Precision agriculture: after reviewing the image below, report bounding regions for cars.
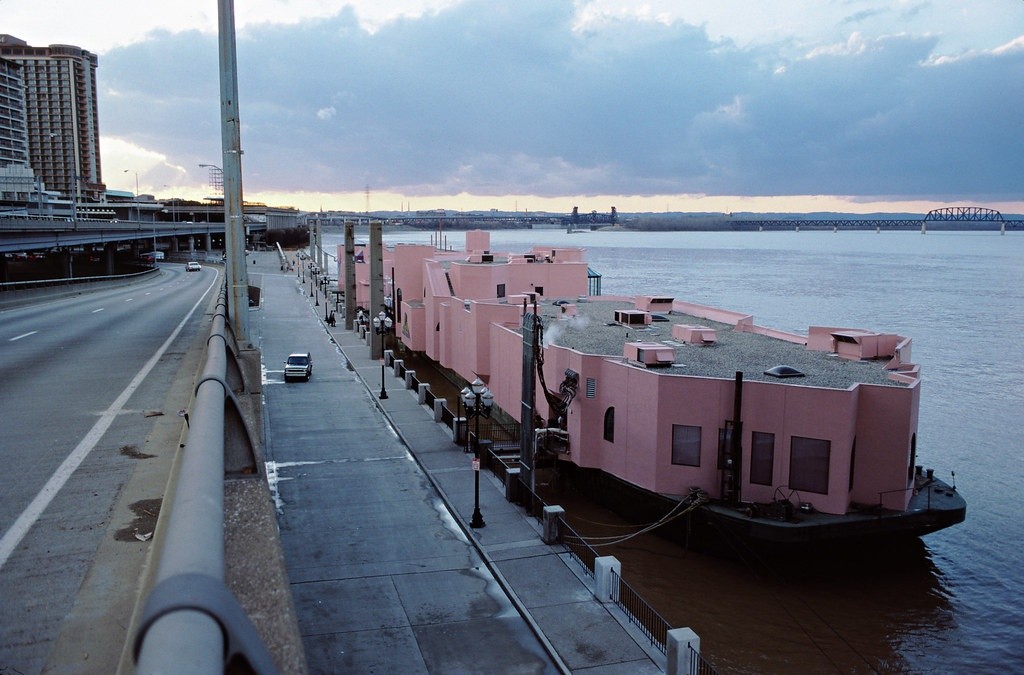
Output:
[184,262,202,272]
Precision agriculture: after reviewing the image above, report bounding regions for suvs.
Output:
[283,352,312,382]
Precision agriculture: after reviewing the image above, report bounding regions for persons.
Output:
[384,306,393,321]
[290,359,300,364]
[298,358,304,365]
[329,310,336,327]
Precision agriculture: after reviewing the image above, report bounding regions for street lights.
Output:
[308,262,315,297]
[373,311,393,399]
[164,184,175,223]
[124,169,142,220]
[50,133,77,222]
[319,274,331,321]
[299,254,307,283]
[152,209,168,264]
[295,251,302,277]
[460,375,495,529]
[312,267,321,306]
